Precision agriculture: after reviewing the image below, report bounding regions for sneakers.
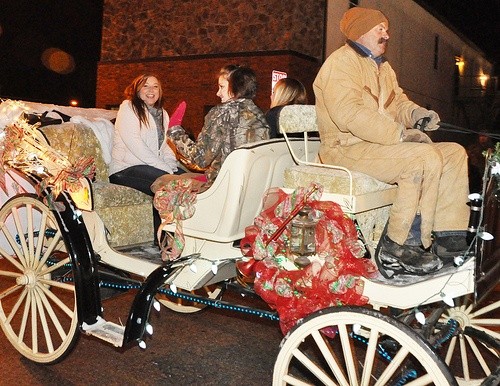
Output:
[379,249,437,274]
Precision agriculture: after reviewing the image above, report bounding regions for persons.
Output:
[108,71,188,250]
[263,78,317,138]
[312,7,474,273]
[152,64,270,193]
[466,129,494,192]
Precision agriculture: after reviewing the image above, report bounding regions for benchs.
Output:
[162,137,321,242]
[279,105,399,214]
[39,123,155,248]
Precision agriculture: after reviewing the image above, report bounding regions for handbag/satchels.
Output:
[155,179,197,220]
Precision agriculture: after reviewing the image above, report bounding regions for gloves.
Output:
[168,101,187,128]
[402,129,432,144]
[412,107,440,131]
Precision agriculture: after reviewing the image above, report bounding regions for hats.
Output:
[340,8,390,43]
